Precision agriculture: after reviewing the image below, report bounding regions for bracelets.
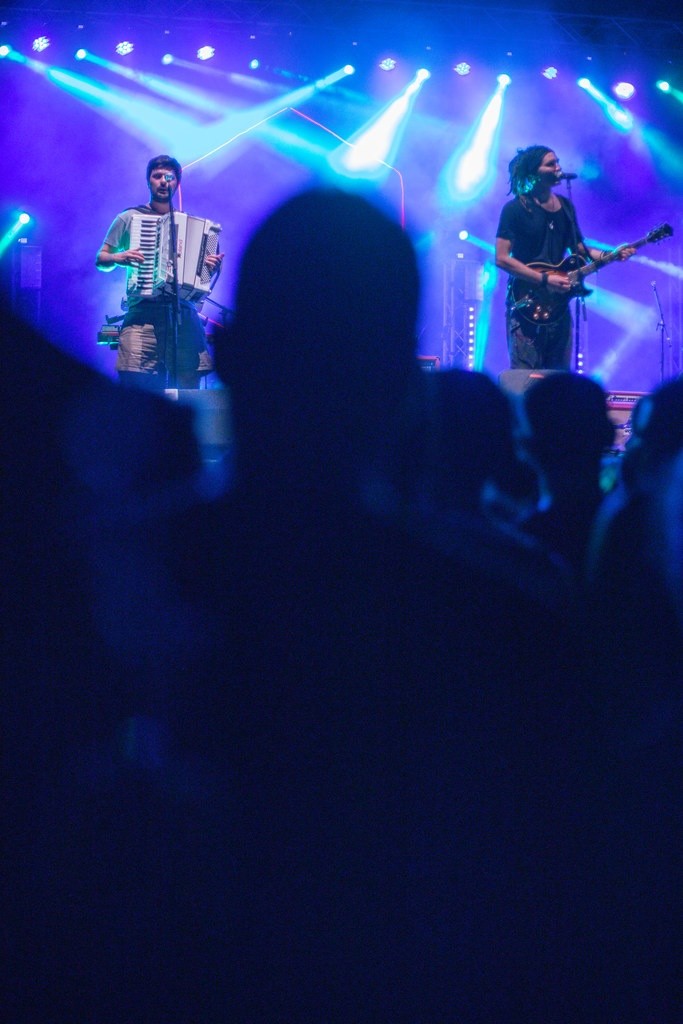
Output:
[542,274,549,284]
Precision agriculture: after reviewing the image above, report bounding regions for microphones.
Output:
[557,172,577,179]
[164,172,174,181]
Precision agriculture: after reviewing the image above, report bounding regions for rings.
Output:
[559,284,562,288]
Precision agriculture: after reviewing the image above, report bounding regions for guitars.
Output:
[511,220,676,325]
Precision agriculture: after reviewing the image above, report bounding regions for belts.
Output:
[141,296,195,310]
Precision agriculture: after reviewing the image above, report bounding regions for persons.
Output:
[95,154,226,399]
[494,144,638,371]
[0,190,683,1021]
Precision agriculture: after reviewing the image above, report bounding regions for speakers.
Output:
[164,388,233,460]
[605,390,650,452]
[497,369,561,397]
